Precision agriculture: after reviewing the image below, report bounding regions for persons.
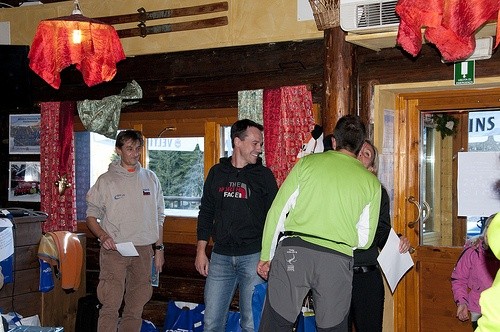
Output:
[85,130,166,332]
[256,115,500,332]
[193,118,278,332]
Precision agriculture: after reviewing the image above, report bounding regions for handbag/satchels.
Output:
[140,319,158,332]
[296,296,317,332]
[164,300,205,332]
[225,311,242,332]
[252,281,268,332]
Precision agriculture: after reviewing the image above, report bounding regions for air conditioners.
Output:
[340,0,400,35]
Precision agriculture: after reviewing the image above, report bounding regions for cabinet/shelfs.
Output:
[0,215,86,332]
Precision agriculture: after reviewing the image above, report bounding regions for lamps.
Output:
[27,0,126,90]
[395,0,500,62]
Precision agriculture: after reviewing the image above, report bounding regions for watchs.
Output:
[155,244,164,251]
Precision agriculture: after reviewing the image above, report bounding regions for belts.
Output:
[354,266,377,274]
